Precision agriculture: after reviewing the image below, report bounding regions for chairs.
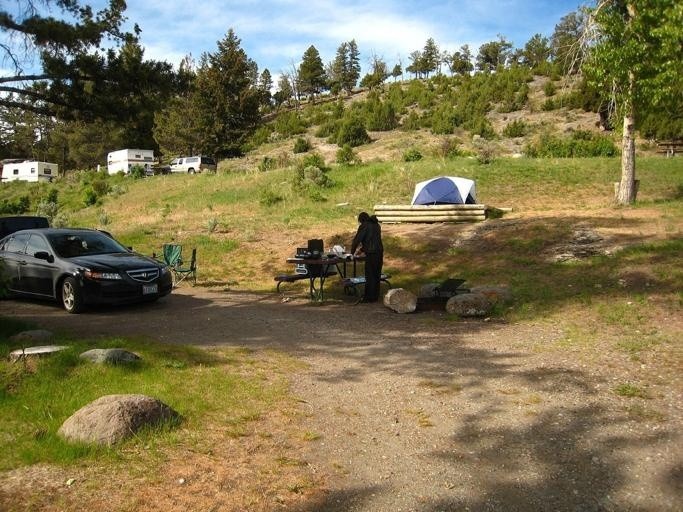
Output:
[173,248,197,289]
[151,243,182,286]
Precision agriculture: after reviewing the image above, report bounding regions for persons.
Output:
[349,211,384,303]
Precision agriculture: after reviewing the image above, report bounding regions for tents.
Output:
[410,175,477,204]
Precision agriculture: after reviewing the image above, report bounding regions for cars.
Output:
[170,156,217,174]
[0,227,171,314]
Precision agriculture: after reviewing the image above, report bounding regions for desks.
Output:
[287,256,366,303]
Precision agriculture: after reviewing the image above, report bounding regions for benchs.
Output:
[337,274,392,299]
[275,272,337,293]
